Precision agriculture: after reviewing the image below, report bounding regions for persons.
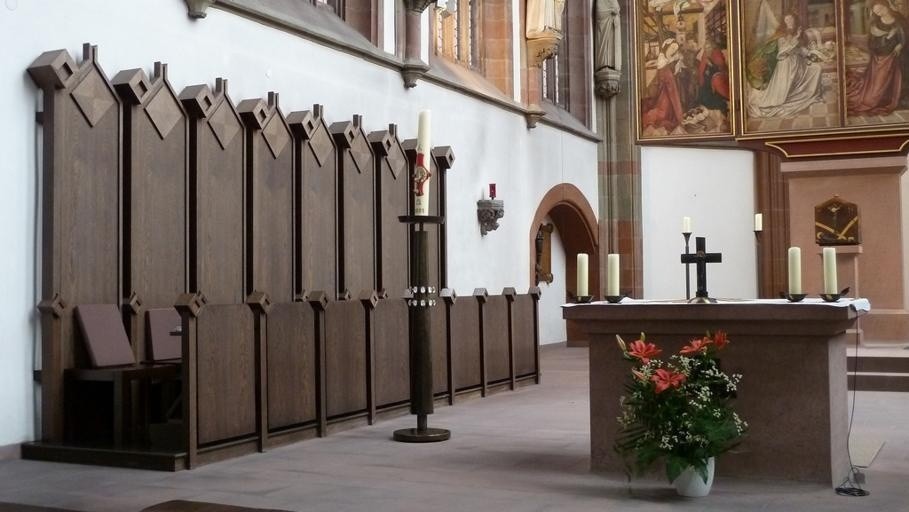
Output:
[637,28,730,130]
[846,0,908,117]
[746,9,825,122]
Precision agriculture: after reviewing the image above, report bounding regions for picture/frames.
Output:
[728,0,844,140]
[835,0,909,134]
[624,1,739,146]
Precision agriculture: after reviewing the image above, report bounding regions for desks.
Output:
[556,292,873,488]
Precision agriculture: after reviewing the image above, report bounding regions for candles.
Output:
[414,105,431,218]
[787,246,804,295]
[823,246,839,295]
[683,215,691,234]
[576,251,590,297]
[606,252,620,296]
[489,183,497,197]
[755,213,762,233]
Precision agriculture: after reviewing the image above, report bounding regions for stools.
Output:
[64,363,175,456]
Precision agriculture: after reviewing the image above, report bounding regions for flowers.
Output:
[594,317,760,485]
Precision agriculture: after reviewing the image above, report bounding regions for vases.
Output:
[667,452,716,498]
